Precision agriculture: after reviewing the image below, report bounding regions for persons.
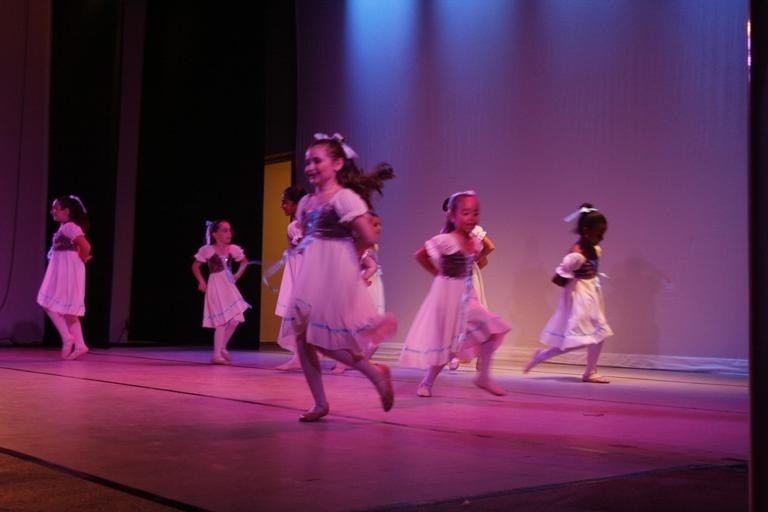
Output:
[523,203,614,384]
[36,195,92,360]
[191,219,252,363]
[274,133,396,421]
[397,191,510,395]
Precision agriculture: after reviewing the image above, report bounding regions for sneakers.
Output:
[221,348,231,361]
[583,372,610,383]
[373,365,392,412]
[68,345,87,360]
[416,382,431,397]
[472,378,506,396]
[298,405,329,421]
[211,357,225,363]
[61,337,74,360]
[448,362,457,369]
[275,345,377,374]
[522,351,540,372]
